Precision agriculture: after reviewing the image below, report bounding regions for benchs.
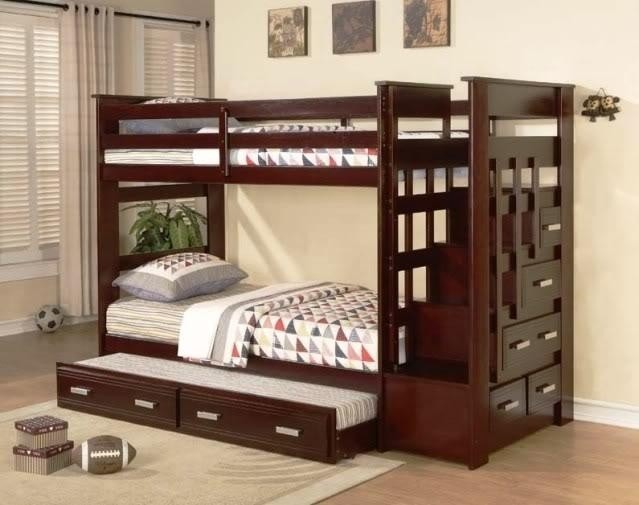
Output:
[55,347,379,465]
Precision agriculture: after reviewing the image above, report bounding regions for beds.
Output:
[89,90,498,471]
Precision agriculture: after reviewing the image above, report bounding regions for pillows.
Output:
[110,249,250,303]
[116,95,241,134]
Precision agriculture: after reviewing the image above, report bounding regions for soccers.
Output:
[34,304,65,334]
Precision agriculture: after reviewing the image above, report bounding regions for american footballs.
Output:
[69,435,137,475]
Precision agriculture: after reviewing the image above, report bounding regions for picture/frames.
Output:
[266,6,309,58]
[331,0,378,55]
[402,0,452,48]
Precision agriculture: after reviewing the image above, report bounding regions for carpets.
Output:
[1,396,407,505]
